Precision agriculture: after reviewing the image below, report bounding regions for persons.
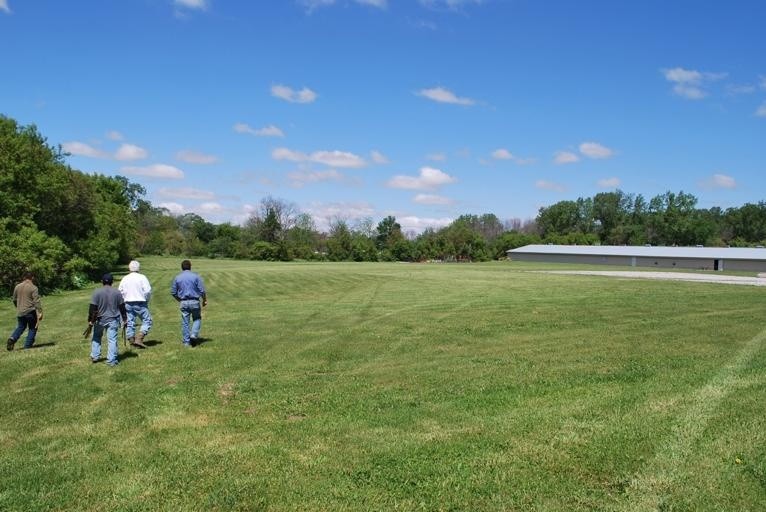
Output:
[118,260,152,348]
[6,272,43,352]
[171,259,207,346]
[88,272,128,366]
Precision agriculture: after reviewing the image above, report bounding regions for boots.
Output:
[128,332,148,349]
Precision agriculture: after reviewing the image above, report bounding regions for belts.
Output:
[180,298,199,301]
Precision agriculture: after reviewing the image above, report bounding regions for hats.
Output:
[102,273,113,282]
[129,260,140,272]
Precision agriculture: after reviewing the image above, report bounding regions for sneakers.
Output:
[7,337,14,351]
